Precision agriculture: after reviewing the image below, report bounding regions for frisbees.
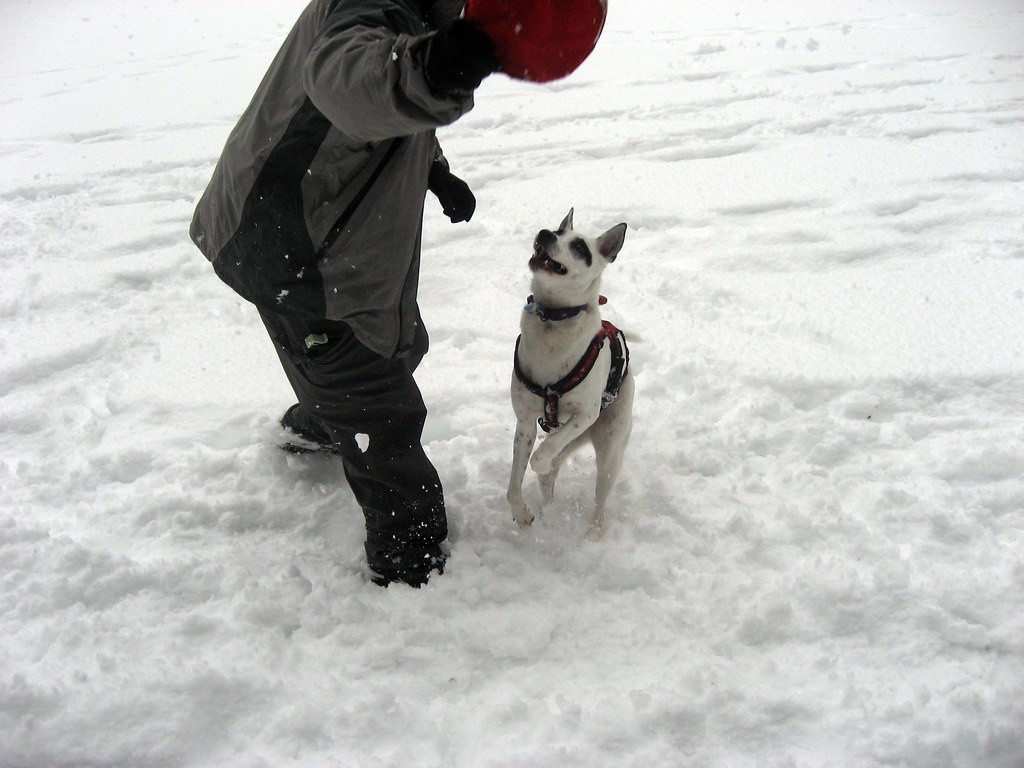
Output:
[466,0,608,84]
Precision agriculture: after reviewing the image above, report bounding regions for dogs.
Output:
[505,205,647,545]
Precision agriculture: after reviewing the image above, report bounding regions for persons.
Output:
[187,0,504,588]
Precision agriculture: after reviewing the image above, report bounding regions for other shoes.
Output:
[276,402,340,455]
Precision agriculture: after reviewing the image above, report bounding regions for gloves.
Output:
[437,173,476,223]
[422,18,504,90]
